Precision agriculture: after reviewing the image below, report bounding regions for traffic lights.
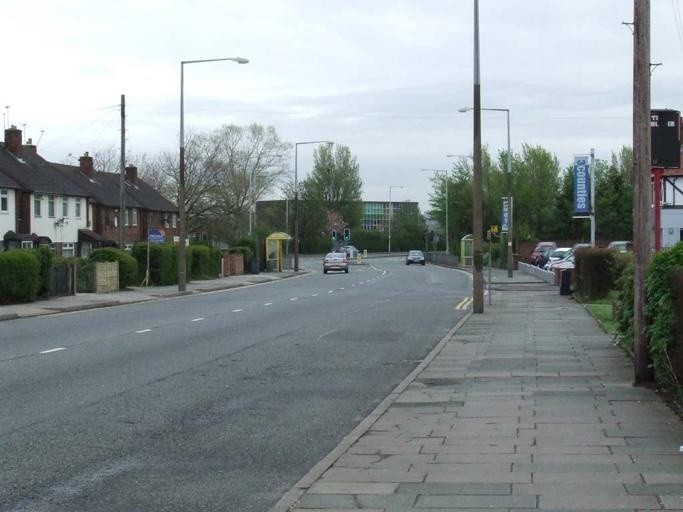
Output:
[344,228,351,240]
[332,230,337,240]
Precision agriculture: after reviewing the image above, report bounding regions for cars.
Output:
[405,250,425,265]
[323,252,348,274]
[530,240,632,272]
[341,246,359,256]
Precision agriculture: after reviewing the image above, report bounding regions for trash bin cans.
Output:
[251,258,260,274]
[560,269,571,295]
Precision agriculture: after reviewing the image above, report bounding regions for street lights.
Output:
[178,56,249,292]
[388,185,404,253]
[457,107,514,278]
[294,140,335,272]
[446,154,473,159]
[249,154,283,235]
[421,168,449,255]
